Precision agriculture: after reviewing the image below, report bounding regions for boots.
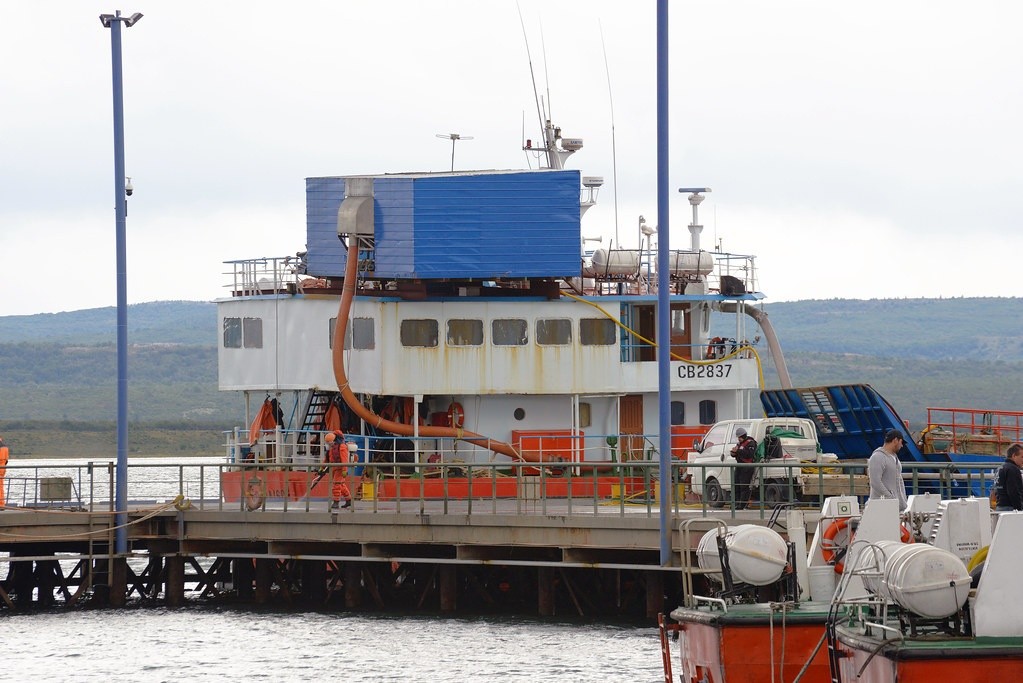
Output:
[341,499,351,508]
[331,501,339,508]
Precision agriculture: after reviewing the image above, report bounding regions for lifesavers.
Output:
[447,402,465,429]
[706,336,722,361]
[823,519,863,574]
[243,476,265,510]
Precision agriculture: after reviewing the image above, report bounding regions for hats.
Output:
[885,429,908,446]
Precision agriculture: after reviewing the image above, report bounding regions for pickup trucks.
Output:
[684,415,868,503]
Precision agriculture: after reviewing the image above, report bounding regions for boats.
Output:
[214,4,1021,506]
[655,490,939,683]
[824,490,1022,683]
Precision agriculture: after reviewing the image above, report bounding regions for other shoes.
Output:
[731,504,748,510]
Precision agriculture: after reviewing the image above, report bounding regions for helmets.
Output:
[325,433,336,442]
[736,428,746,437]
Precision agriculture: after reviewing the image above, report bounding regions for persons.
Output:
[313,432,352,509]
[0,439,9,508]
[868,430,908,511]
[728,428,758,509]
[994,443,1023,512]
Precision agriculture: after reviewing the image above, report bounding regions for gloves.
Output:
[342,471,347,478]
[318,467,323,474]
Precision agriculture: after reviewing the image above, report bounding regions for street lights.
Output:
[101,9,145,605]
